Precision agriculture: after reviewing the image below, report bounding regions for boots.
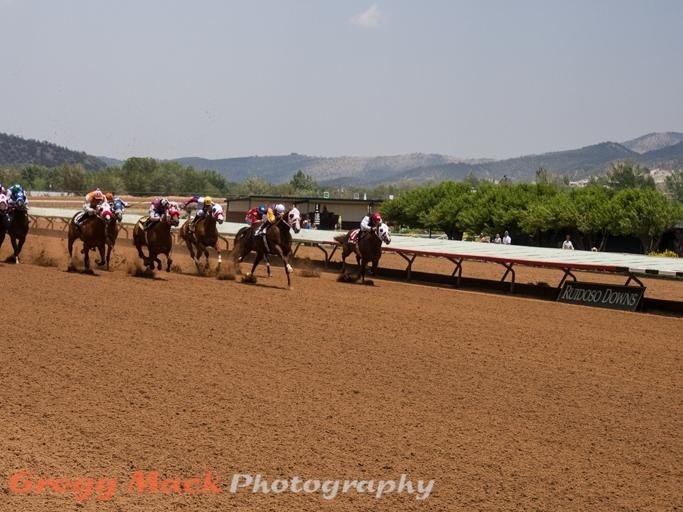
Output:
[76,212,89,227]
[258,223,266,235]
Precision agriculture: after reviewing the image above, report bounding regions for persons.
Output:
[0,182,30,222]
[302,218,317,231]
[74,190,130,233]
[288,204,300,222]
[476,230,511,263]
[560,234,576,250]
[333,222,343,230]
[347,211,382,243]
[148,194,287,236]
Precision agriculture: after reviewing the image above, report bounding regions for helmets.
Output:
[258,204,266,215]
[106,192,113,201]
[276,204,285,214]
[204,196,212,206]
[162,199,169,208]
[14,184,21,192]
[370,213,381,225]
[94,190,103,200]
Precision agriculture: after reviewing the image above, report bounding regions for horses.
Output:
[233,204,302,291]
[65,202,113,273]
[179,203,227,277]
[77,198,129,270]
[0,185,29,265]
[132,200,181,273]
[333,220,391,286]
